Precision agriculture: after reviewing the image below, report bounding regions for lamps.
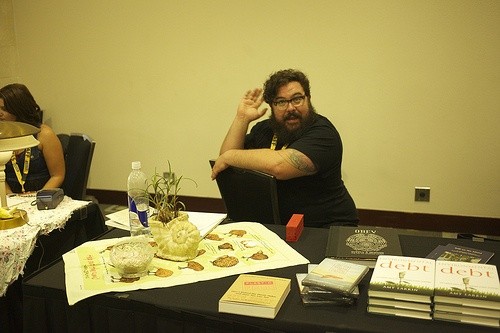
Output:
[0,121,40,230]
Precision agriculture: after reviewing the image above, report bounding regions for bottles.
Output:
[127,162,150,237]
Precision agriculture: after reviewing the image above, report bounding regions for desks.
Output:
[0,195,500,333]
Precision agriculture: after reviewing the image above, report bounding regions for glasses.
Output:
[271,93,306,106]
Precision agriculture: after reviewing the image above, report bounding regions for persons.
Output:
[0,83,66,197]
[211,69,359,229]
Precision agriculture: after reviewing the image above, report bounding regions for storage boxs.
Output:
[286,214,304,242]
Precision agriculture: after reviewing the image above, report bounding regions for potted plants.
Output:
[126,159,200,262]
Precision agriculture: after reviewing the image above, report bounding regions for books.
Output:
[366,242,500,329]
[325,225,403,268]
[296,257,369,305]
[105,204,158,232]
[219,273,291,320]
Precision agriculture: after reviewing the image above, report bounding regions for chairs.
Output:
[57,132,96,201]
[208,160,279,225]
